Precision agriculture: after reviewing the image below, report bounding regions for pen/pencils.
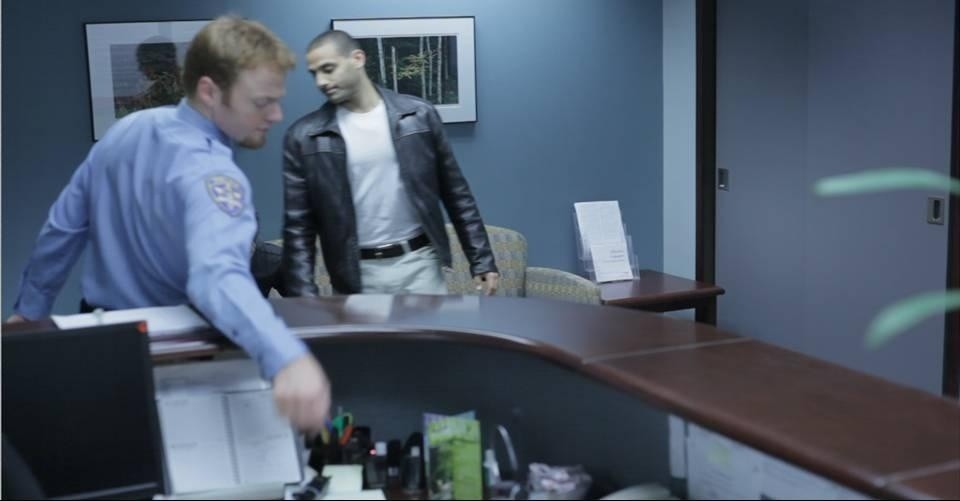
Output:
[93,307,105,326]
[320,406,353,446]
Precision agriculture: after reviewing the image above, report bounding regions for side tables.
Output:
[593,268,726,329]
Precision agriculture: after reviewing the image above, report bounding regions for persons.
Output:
[129,33,184,110]
[280,31,500,300]
[7,11,334,438]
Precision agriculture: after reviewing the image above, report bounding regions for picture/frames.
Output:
[84,18,219,145]
[330,15,478,125]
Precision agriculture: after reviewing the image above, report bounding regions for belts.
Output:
[359,233,430,261]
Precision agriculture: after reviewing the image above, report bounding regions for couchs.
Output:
[264,220,603,312]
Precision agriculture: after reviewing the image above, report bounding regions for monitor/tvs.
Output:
[2,319,174,501]
